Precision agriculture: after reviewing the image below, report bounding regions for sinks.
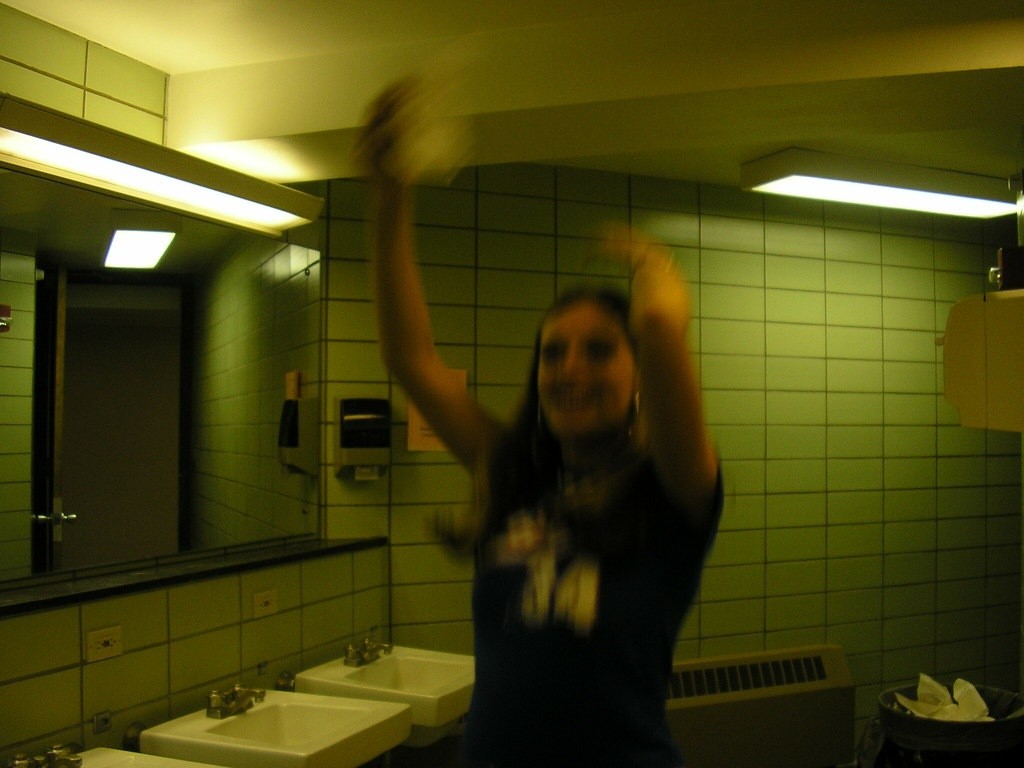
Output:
[137,686,414,767]
[73,745,224,767]
[290,640,480,751]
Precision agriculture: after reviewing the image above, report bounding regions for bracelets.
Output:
[630,246,663,273]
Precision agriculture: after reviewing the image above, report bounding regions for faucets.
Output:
[205,683,267,721]
[343,635,392,668]
[8,740,84,768]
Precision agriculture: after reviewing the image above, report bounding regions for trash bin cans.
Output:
[855,680,1024,768]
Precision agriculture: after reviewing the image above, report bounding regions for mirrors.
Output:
[0,168,320,583]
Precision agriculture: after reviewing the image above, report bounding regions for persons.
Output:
[355,72,724,768]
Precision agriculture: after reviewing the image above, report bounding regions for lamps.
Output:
[741,147,1017,220]
[0,91,326,239]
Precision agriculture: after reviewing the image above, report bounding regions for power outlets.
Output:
[253,589,278,621]
[85,625,122,663]
